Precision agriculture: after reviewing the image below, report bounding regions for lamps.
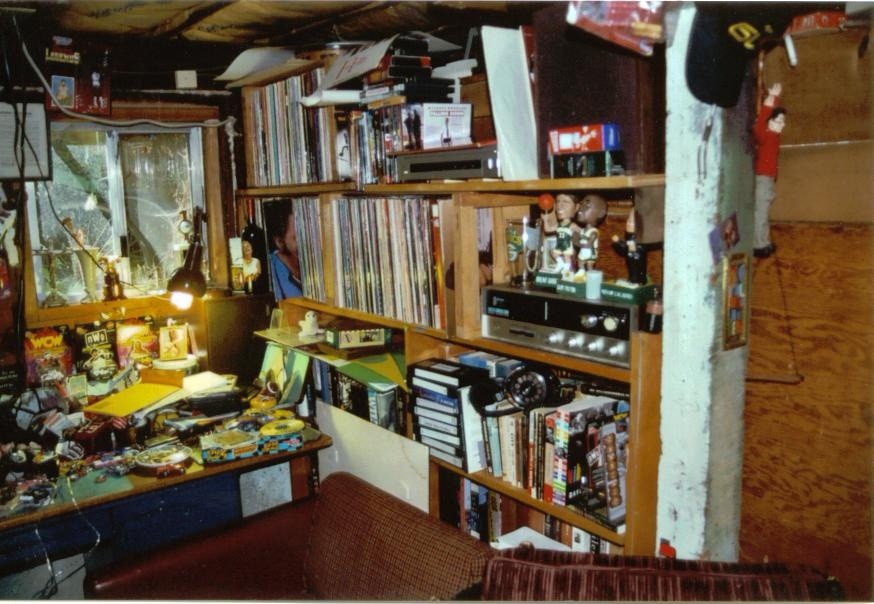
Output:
[167,205,233,311]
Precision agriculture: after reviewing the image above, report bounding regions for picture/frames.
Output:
[720,252,749,350]
[159,324,187,361]
[0,97,54,183]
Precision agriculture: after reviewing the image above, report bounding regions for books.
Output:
[307,358,404,435]
[247,40,472,188]
[248,197,493,331]
[406,357,630,558]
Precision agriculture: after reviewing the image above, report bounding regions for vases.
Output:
[71,247,103,304]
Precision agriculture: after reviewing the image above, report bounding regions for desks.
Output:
[0,382,336,579]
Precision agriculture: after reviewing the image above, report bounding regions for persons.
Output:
[541,192,608,284]
[264,200,303,300]
[752,82,787,259]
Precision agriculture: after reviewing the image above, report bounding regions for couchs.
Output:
[484,552,847,602]
[85,471,499,600]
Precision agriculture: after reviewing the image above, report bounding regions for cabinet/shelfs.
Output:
[239,54,665,560]
